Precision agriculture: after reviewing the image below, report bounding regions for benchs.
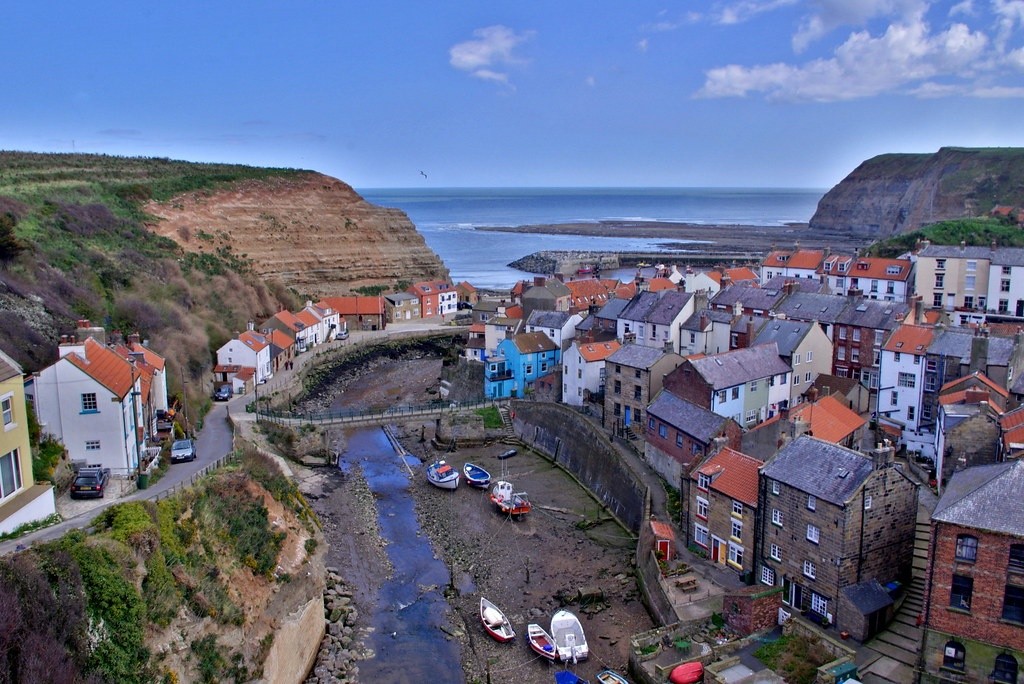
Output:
[682,585,699,593]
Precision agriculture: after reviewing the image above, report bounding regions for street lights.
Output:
[182,373,188,439]
[131,392,141,473]
[201,366,204,392]
[255,384,262,423]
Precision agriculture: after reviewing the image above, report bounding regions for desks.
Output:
[677,575,696,587]
[677,641,691,655]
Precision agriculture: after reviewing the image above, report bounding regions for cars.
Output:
[70,467,109,500]
[170,439,196,464]
[457,302,474,309]
[215,385,232,401]
[336,332,349,340]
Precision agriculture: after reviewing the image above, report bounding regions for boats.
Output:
[596,671,628,684]
[554,670,590,684]
[528,624,556,660]
[490,458,531,514]
[480,596,516,643]
[578,261,593,275]
[636,263,651,268]
[427,460,459,488]
[550,609,588,664]
[670,661,704,684]
[463,463,491,488]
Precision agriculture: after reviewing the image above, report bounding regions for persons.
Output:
[974,321,989,338]
[284,361,289,370]
[289,360,294,370]
[938,320,947,327]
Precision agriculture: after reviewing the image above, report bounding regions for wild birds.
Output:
[420,171,427,179]
